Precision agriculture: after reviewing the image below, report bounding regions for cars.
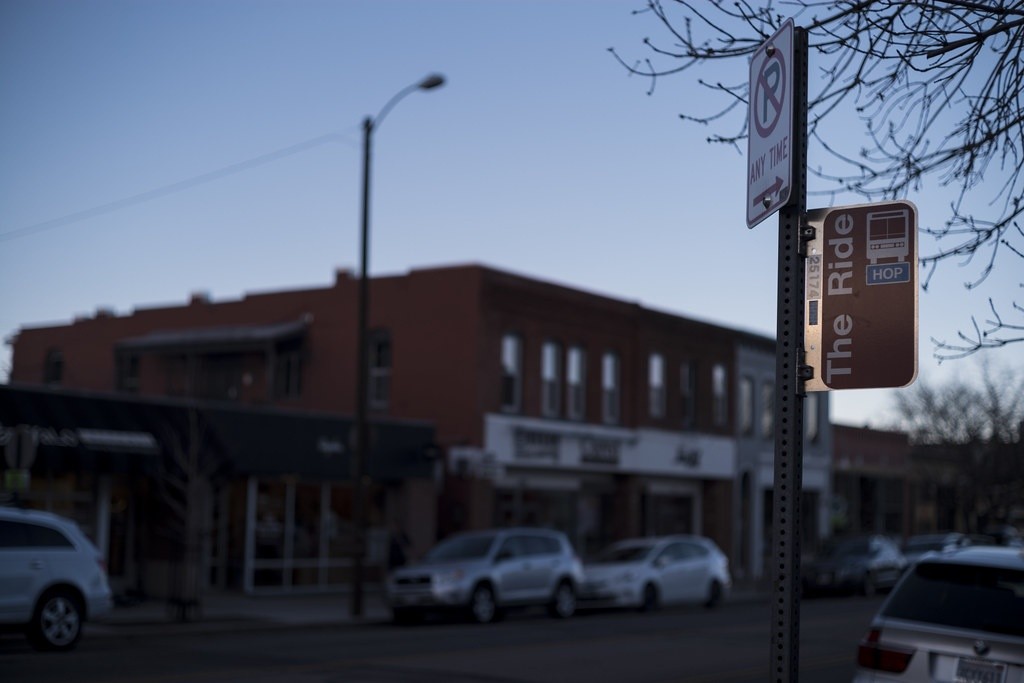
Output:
[584,535,731,618]
[798,526,1024,594]
[388,525,591,624]
[0,506,115,653]
[851,546,1024,683]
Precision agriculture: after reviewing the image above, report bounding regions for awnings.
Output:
[0,385,436,483]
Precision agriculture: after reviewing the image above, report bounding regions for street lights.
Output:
[349,72,446,616]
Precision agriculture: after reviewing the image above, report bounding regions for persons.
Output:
[381,519,411,586]
